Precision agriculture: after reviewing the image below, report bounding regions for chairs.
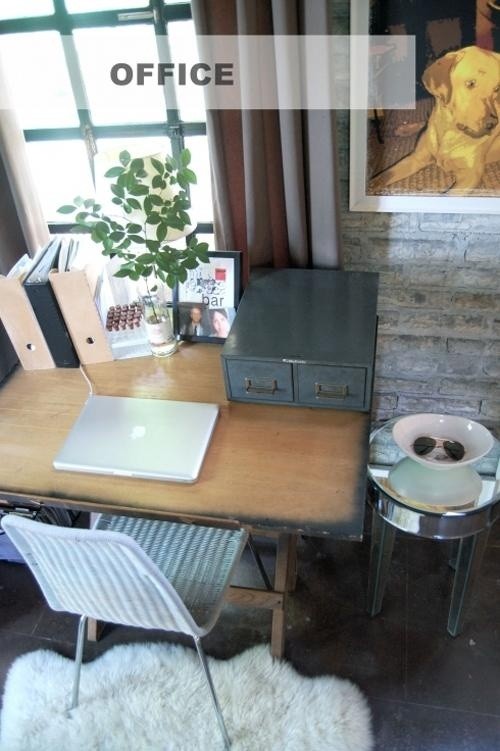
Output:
[0,513,273,751]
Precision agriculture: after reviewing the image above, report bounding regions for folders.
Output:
[0,237,114,371]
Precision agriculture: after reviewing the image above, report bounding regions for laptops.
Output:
[53,395,219,485]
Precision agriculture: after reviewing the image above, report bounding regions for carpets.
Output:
[0,644,377,751]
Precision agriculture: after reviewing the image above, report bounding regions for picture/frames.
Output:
[172,250,241,344]
[350,0,500,215]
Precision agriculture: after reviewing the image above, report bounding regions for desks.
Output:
[0,315,377,663]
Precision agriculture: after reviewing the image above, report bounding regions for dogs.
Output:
[368,44,499,197]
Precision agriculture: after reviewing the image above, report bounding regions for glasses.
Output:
[414,438,464,460]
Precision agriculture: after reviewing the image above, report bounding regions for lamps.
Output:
[93,146,197,307]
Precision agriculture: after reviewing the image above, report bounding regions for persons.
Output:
[208,309,231,338]
[183,307,206,336]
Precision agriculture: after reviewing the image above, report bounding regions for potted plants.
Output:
[56,148,211,358]
